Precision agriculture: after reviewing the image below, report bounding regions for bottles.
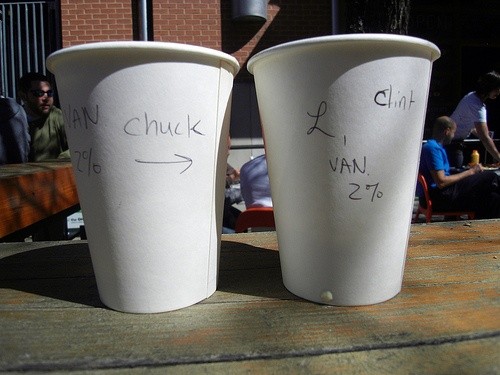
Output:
[471,150,480,165]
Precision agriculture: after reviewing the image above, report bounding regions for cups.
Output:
[246,34,441,306]
[45,41,240,313]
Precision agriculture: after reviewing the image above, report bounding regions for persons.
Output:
[444,73,500,171]
[17,71,74,162]
[221,136,245,232]
[415,117,500,220]
[239,155,277,231]
[0,96,31,165]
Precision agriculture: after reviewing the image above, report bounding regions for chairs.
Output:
[234,207,275,234]
[414,175,476,224]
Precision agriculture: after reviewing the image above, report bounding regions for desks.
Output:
[1,159,79,242]
[0,219,499,375]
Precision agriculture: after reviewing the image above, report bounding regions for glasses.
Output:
[30,88,53,97]
